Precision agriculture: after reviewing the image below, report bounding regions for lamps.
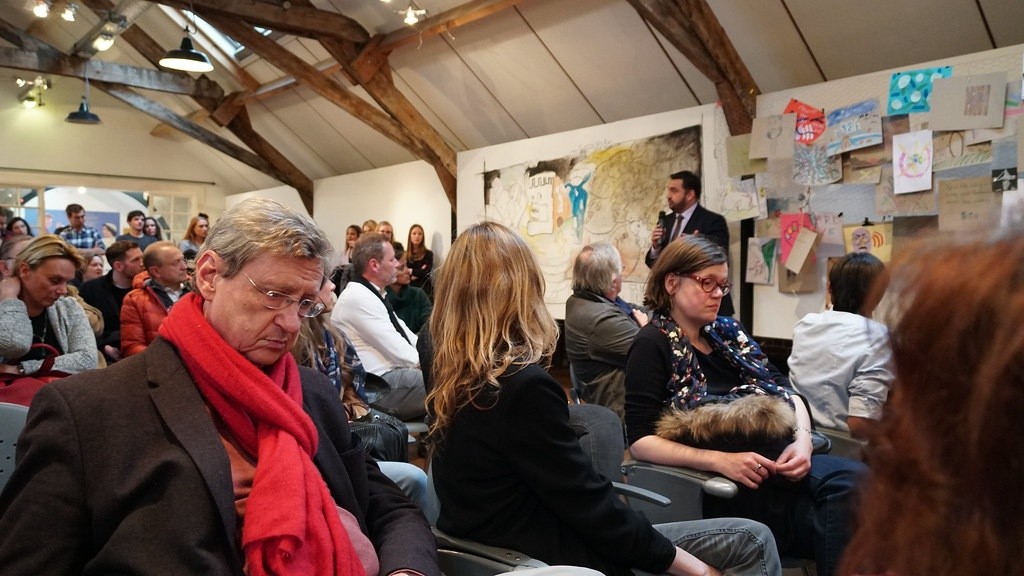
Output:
[33,0,55,17]
[91,33,114,51]
[16,76,52,107]
[392,1,426,25]
[60,4,80,22]
[64,76,102,124]
[158,24,214,73]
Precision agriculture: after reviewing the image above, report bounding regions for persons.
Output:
[644,170,735,318]
[0,196,440,576]
[300,273,439,526]
[625,234,868,576]
[425,221,782,576]
[838,223,1024,576]
[330,234,430,421]
[0,203,211,377]
[564,242,654,448]
[787,252,897,438]
[337,219,433,333]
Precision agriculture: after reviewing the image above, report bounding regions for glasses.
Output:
[165,258,188,266]
[240,270,327,317]
[686,275,732,296]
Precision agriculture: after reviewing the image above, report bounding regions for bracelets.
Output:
[792,427,813,442]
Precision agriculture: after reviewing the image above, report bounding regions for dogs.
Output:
[654,393,796,450]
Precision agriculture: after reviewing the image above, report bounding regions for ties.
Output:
[672,215,684,241]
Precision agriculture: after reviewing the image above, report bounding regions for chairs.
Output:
[0,402,31,493]
[432,360,873,576]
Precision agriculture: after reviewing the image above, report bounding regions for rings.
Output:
[755,464,761,473]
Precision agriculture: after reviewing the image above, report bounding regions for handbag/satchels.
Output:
[0,344,68,407]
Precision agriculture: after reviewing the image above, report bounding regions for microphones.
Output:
[657,211,665,244]
